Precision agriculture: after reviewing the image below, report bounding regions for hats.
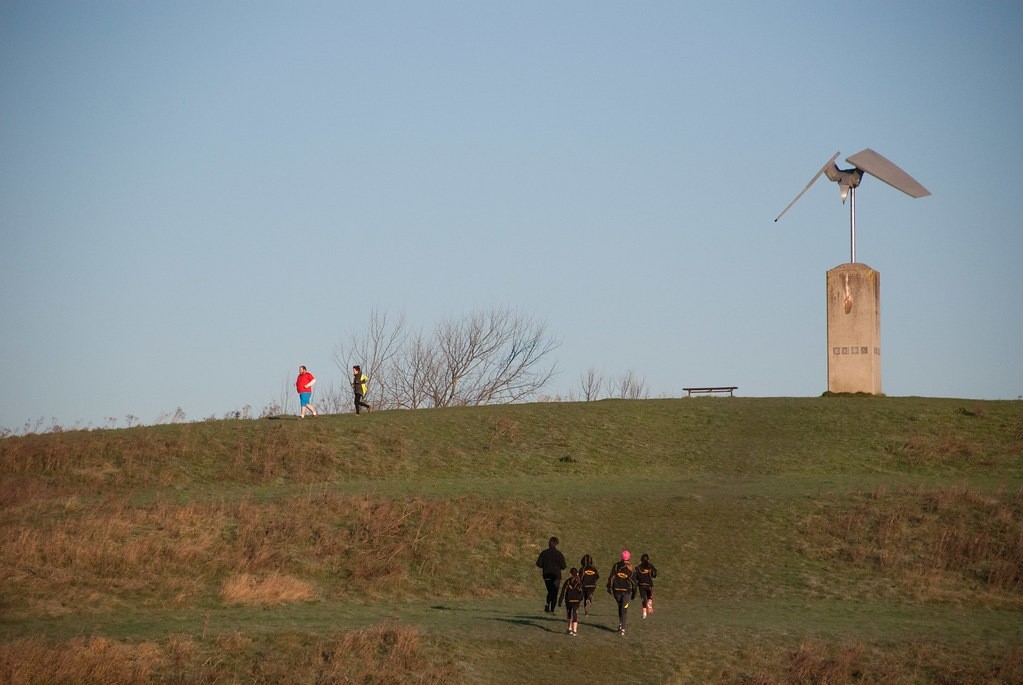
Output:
[621,551,631,561]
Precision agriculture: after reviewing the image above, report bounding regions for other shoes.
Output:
[618,624,626,635]
[550,611,557,616]
[585,613,589,618]
[566,629,573,635]
[367,405,372,413]
[312,411,318,417]
[586,599,591,608]
[296,416,304,420]
[352,414,360,416]
[573,632,578,636]
[545,604,547,613]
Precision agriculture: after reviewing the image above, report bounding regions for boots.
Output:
[647,599,654,615]
[642,607,647,620]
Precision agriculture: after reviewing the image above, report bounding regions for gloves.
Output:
[631,590,637,600]
[606,587,611,593]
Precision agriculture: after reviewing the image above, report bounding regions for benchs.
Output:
[682,387,738,397]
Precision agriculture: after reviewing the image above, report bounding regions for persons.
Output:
[579,554,599,618]
[536,536,567,616]
[294,366,319,419]
[558,567,582,637]
[352,365,373,416]
[634,554,657,619]
[607,550,637,636]
